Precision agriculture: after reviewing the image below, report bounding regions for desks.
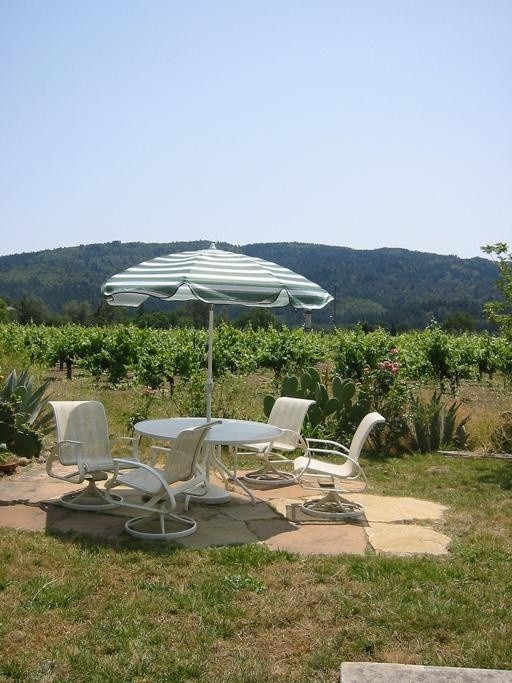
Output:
[134,418,283,511]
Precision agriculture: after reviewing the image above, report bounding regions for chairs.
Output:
[229,397,316,484]
[104,420,222,540]
[293,412,386,518]
[46,401,139,510]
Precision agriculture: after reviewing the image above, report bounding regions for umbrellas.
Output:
[100,241,334,485]
